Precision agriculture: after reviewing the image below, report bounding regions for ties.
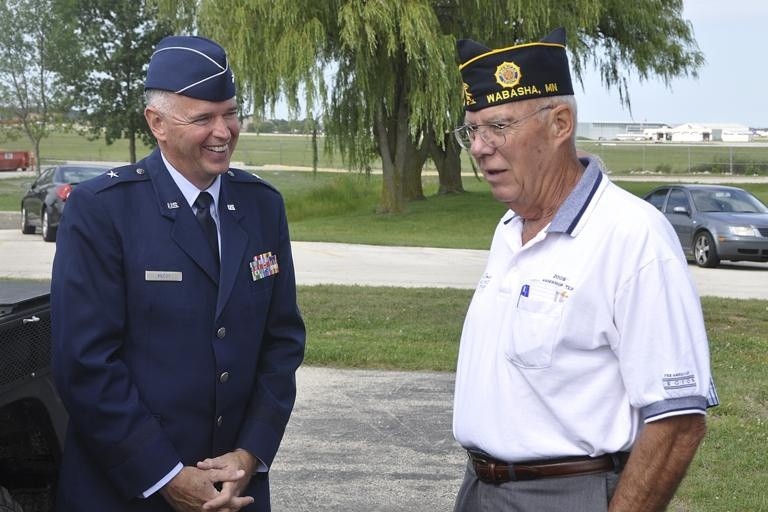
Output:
[191,191,224,274]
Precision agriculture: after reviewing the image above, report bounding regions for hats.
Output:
[452,24,574,112]
[143,33,235,102]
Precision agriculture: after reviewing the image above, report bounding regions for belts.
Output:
[466,444,626,485]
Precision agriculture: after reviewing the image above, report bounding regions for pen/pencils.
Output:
[515,284,531,307]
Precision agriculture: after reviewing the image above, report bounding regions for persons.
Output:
[453,27,719,512]
[50,37,305,512]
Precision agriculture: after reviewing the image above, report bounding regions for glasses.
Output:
[451,106,558,150]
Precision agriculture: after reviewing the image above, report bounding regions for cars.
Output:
[644,184,768,268]
[17,164,111,243]
